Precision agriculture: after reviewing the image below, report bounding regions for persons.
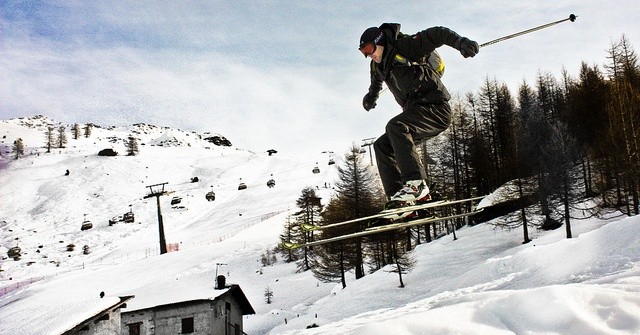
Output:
[359,22,480,225]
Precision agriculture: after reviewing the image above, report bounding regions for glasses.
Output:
[358,41,377,58]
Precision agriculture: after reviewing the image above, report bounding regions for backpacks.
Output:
[372,50,445,80]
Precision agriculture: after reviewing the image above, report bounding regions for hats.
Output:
[360,27,387,46]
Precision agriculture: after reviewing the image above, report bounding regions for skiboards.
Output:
[282,194,486,250]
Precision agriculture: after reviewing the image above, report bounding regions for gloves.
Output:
[363,88,379,112]
[456,37,479,58]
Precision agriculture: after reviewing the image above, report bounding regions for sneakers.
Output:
[385,178,432,209]
[378,209,419,223]
